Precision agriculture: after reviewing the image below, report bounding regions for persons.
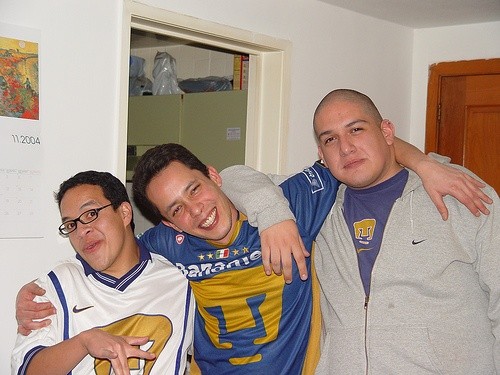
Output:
[215,89,499,374]
[15,136,493,374]
[10,170,198,375]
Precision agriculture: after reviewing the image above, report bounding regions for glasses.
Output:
[58,203,120,235]
